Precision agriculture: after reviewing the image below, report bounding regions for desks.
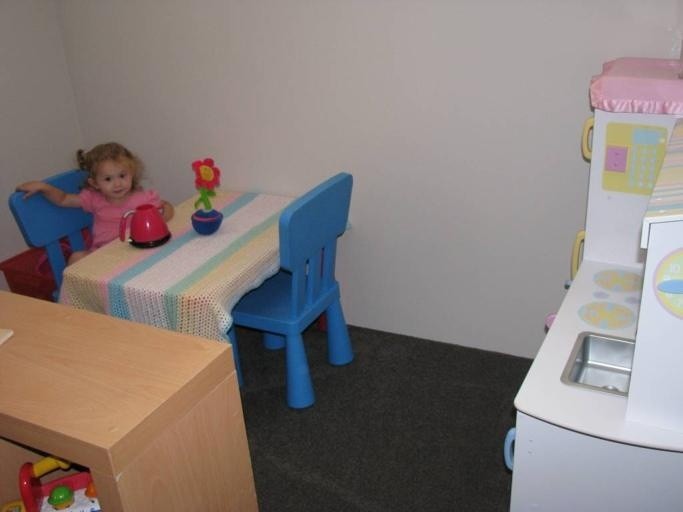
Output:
[62,191,297,342]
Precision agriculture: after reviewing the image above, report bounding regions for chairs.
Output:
[225,170,353,408]
[8,170,95,302]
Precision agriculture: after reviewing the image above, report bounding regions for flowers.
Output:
[190,158,223,212]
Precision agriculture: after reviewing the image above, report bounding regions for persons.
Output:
[14,142,175,265]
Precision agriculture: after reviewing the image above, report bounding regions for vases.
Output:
[191,208,224,236]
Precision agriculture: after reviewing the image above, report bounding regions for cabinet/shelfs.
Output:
[0,289,262,512]
